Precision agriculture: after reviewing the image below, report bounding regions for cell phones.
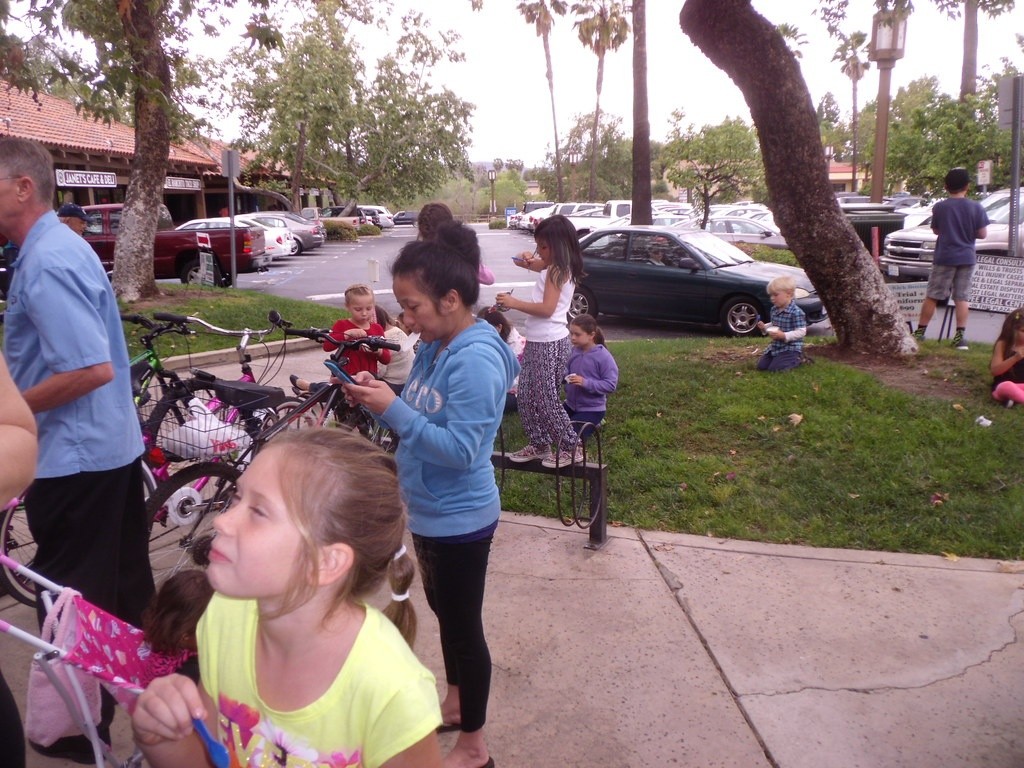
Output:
[324,360,357,385]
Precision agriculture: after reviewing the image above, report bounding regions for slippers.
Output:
[434,713,466,733]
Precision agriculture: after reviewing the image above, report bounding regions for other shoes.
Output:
[1003,398,1016,408]
[290,374,304,389]
[28,728,113,762]
[910,330,926,343]
[950,339,968,351]
[292,387,310,399]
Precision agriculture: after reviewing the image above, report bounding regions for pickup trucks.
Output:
[81,202,272,288]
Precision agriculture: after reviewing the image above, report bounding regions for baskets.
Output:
[128,378,270,461]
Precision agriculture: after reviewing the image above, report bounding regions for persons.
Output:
[323,284,391,435]
[290,305,415,398]
[0,137,156,765]
[142,569,214,684]
[132,430,444,768]
[562,314,618,438]
[341,222,522,768]
[57,202,95,237]
[477,307,525,415]
[394,311,422,354]
[494,215,588,468]
[758,276,814,372]
[912,169,990,350]
[647,243,664,265]
[0,355,38,768]
[988,307,1024,408]
[418,203,494,285]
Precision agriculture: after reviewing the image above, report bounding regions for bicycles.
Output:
[0,310,402,609]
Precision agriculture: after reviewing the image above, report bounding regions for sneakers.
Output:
[542,451,585,467]
[511,445,553,461]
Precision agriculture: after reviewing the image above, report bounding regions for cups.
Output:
[564,373,577,385]
[496,291,512,313]
[767,327,780,336]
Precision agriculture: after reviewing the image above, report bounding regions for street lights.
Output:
[487,170,496,217]
[867,9,908,203]
[823,142,833,178]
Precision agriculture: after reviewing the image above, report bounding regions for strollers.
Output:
[0,497,200,768]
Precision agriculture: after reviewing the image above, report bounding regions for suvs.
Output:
[878,186,1024,281]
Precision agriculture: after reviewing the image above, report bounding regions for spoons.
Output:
[193,717,229,768]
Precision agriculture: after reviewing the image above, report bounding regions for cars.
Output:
[566,224,829,338]
[508,191,993,250]
[174,205,421,260]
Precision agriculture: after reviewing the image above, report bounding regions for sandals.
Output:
[797,352,815,365]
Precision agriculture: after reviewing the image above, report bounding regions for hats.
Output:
[57,202,96,223]
[945,167,973,187]
